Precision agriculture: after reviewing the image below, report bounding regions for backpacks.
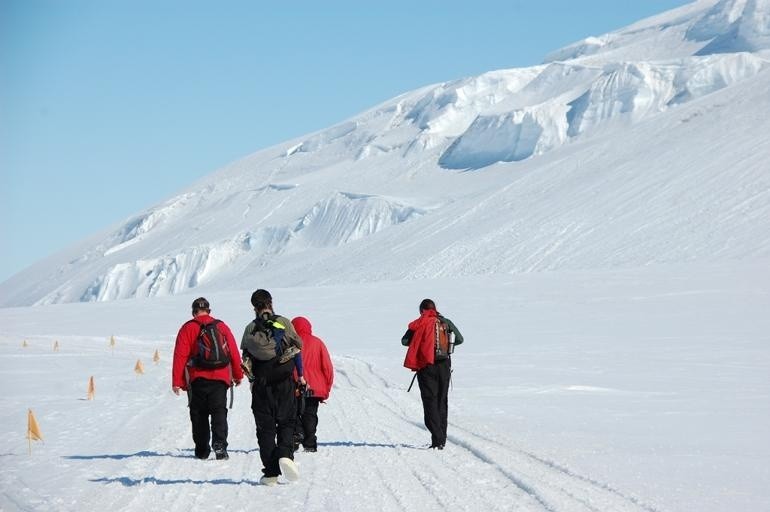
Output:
[192,319,230,370]
[433,316,455,360]
[241,315,302,383]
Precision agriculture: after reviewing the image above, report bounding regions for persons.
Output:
[401,299,463,449]
[290,316,333,452]
[240,289,310,486]
[172,297,243,460]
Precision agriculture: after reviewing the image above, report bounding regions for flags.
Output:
[153,350,160,364]
[134,359,144,375]
[27,412,43,440]
[86,375,95,398]
[110,336,116,347]
[54,340,61,351]
[22,341,27,348]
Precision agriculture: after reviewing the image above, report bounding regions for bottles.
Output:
[448,331,457,354]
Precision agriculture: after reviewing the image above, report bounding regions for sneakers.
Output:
[430,440,446,449]
[212,441,228,459]
[261,458,298,485]
[294,433,317,453]
